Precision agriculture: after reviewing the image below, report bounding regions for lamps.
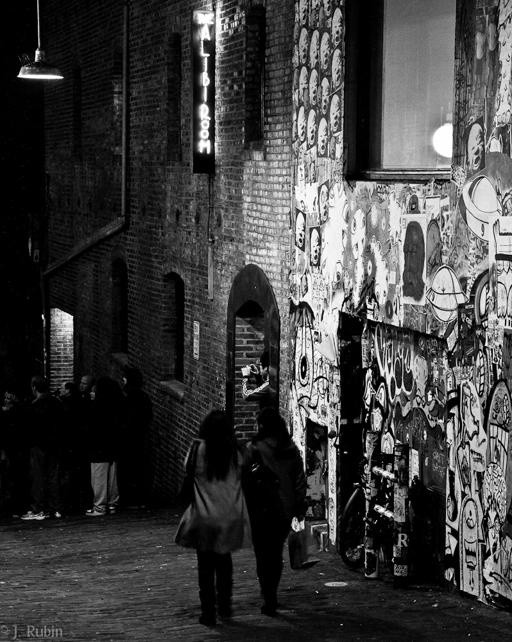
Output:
[17,1,65,82]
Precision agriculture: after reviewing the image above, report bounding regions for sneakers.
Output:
[109,505,116,513]
[260,603,278,610]
[85,509,107,517]
[44,511,62,519]
[21,511,45,521]
[199,615,216,625]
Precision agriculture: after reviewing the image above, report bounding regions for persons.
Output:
[241,412,308,616]
[0,370,152,522]
[174,411,252,629]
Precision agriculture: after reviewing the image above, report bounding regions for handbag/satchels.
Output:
[173,506,234,552]
[288,530,320,570]
